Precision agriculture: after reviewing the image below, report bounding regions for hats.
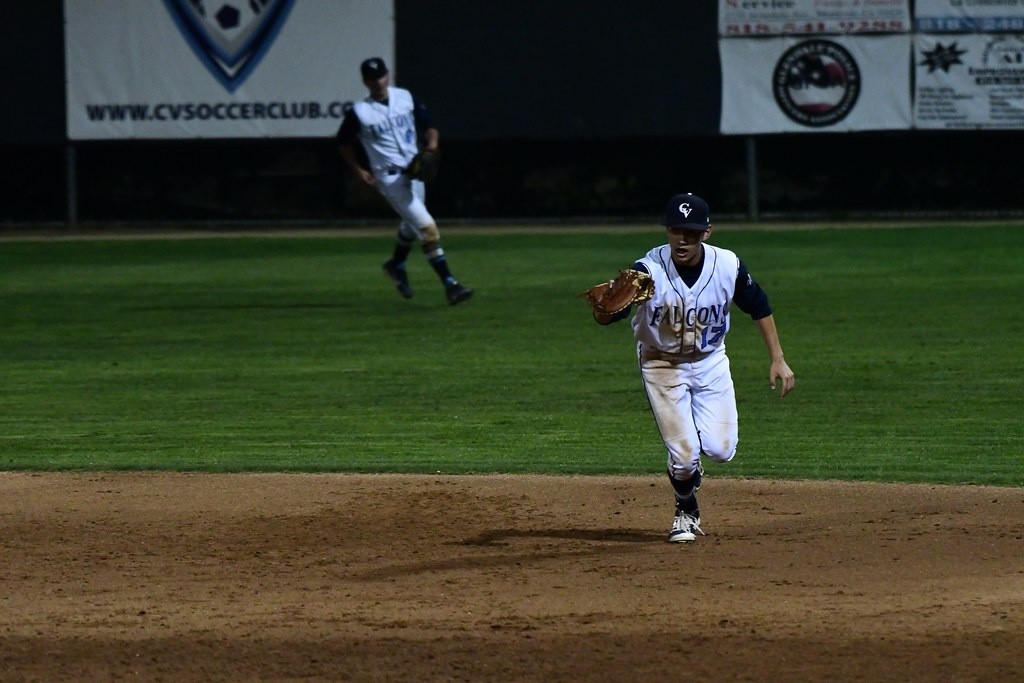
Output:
[660,194,709,230]
[361,57,389,77]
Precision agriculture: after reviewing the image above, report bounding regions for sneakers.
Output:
[668,508,705,542]
[693,460,704,490]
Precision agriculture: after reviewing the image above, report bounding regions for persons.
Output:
[593,194,795,544]
[336,58,474,306]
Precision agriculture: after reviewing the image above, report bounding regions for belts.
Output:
[645,347,714,364]
[388,169,406,176]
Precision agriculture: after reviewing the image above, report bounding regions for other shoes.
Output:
[384,261,412,299]
[446,280,472,305]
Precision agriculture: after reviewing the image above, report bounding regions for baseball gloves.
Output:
[403,149,442,182]
[582,268,656,316]
